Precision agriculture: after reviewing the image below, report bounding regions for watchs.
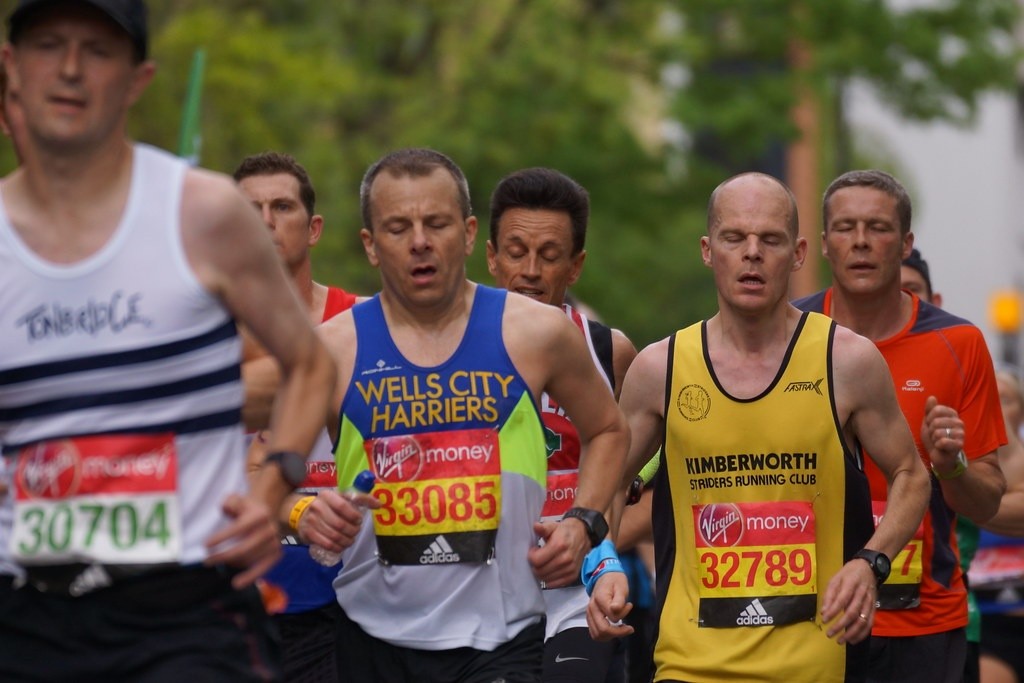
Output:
[563,508,609,548]
[847,549,891,586]
[266,451,306,489]
[626,476,644,505]
[930,452,967,481]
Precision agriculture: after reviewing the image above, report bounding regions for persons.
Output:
[0,0,340,683]
[227,153,1024,683]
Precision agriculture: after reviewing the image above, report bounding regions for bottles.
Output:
[309,470,375,567]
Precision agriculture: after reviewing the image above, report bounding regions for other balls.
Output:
[988,291,1023,334]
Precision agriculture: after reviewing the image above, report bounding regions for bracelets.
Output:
[288,496,316,532]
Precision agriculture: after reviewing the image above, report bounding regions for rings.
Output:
[541,581,546,590]
[946,429,952,438]
[860,614,869,622]
